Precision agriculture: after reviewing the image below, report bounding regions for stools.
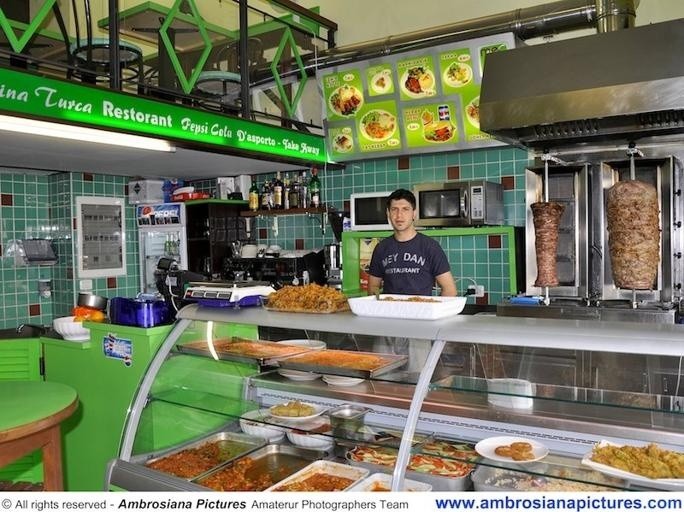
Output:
[70,38,146,99]
[196,69,244,112]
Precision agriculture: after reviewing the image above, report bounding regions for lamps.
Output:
[0,114,175,153]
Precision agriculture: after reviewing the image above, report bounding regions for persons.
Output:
[368,189,457,372]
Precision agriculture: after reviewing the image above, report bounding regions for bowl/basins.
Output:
[239,408,285,441]
[286,418,337,451]
[51,315,90,341]
[77,292,108,310]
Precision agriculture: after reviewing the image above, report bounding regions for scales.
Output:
[183,282,275,308]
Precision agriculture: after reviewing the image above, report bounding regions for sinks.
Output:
[0,326,42,339]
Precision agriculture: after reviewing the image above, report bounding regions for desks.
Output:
[0,383,78,490]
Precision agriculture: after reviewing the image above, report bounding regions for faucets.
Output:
[17,323,47,336]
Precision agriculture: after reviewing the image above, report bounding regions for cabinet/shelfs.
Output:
[40,323,258,491]
[342,226,523,298]
[241,205,338,222]
[108,305,684,493]
[185,200,249,276]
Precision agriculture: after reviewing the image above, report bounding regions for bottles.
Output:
[343,217,349,232]
[177,231,180,255]
[439,105,450,122]
[311,167,321,206]
[262,179,274,209]
[249,181,259,211]
[285,171,311,209]
[274,170,283,209]
[339,264,343,280]
[164,234,170,253]
[170,233,176,255]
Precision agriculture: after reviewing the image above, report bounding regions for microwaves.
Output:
[349,190,428,230]
[412,179,505,230]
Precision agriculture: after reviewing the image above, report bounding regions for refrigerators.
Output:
[135,202,188,300]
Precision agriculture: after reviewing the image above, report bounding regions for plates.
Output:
[262,306,350,316]
[331,133,353,155]
[261,459,369,491]
[345,428,484,490]
[358,109,397,143]
[421,120,457,144]
[328,84,363,118]
[347,294,467,320]
[370,73,392,94]
[470,453,630,491]
[474,436,549,465]
[175,336,316,365]
[240,243,281,258]
[189,443,329,490]
[321,374,364,387]
[464,96,480,128]
[274,339,326,381]
[143,431,269,483]
[344,471,433,490]
[267,401,327,420]
[399,65,435,100]
[581,438,684,491]
[443,62,472,88]
[274,348,408,379]
